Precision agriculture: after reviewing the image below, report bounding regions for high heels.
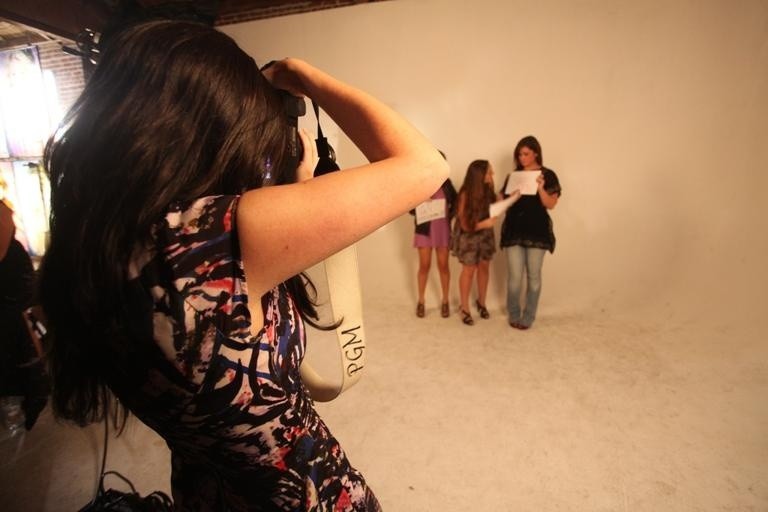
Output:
[475,299,489,319]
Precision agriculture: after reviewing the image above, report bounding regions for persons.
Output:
[449,160,504,325]
[410,151,457,317]
[500,136,561,330]
[0,201,49,431]
[36,17,451,512]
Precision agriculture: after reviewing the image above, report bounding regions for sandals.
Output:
[459,305,474,325]
[416,301,425,318]
[441,301,449,317]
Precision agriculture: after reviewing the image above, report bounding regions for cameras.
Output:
[259,86,306,190]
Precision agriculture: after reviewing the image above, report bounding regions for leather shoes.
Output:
[509,322,528,330]
